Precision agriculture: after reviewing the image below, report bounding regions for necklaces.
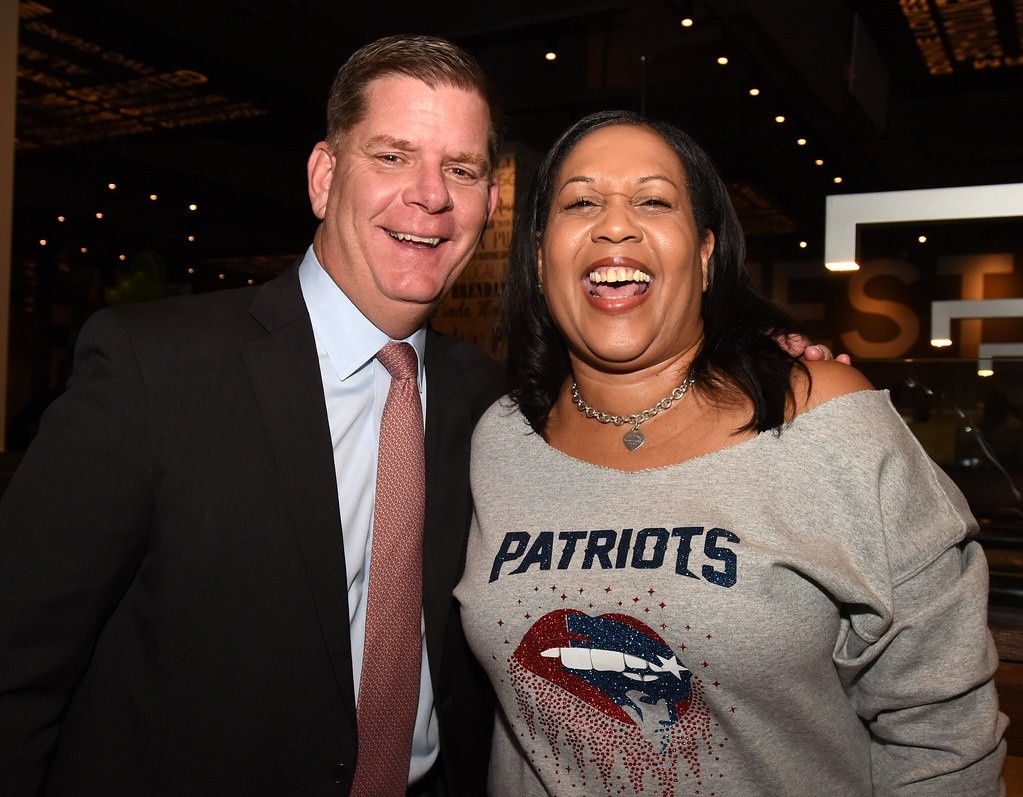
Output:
[570,371,697,452]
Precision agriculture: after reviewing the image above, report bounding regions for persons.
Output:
[451,108,1009,796]
[887,377,1022,511]
[1,35,507,797]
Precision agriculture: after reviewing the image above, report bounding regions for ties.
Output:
[350,342,426,797]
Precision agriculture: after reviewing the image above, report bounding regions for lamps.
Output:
[824,183,1023,377]
